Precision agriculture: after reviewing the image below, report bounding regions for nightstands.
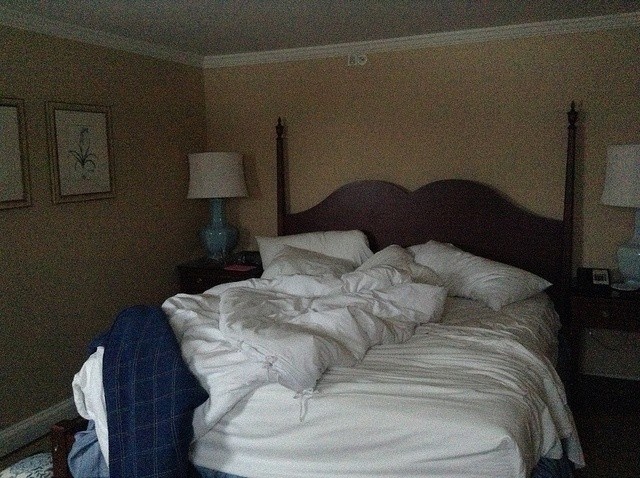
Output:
[565,287,640,403]
[176,254,261,292]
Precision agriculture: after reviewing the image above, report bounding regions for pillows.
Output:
[354,245,443,286]
[260,246,356,281]
[413,240,553,311]
[253,229,374,269]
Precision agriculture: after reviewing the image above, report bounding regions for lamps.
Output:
[184,151,249,260]
[601,144,640,289]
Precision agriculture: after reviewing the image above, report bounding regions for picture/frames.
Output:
[0,96,32,210]
[44,101,116,204]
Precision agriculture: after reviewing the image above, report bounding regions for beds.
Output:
[51,100,587,476]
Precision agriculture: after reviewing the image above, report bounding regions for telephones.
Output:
[577,267,610,289]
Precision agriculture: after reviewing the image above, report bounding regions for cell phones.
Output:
[592,269,610,285]
